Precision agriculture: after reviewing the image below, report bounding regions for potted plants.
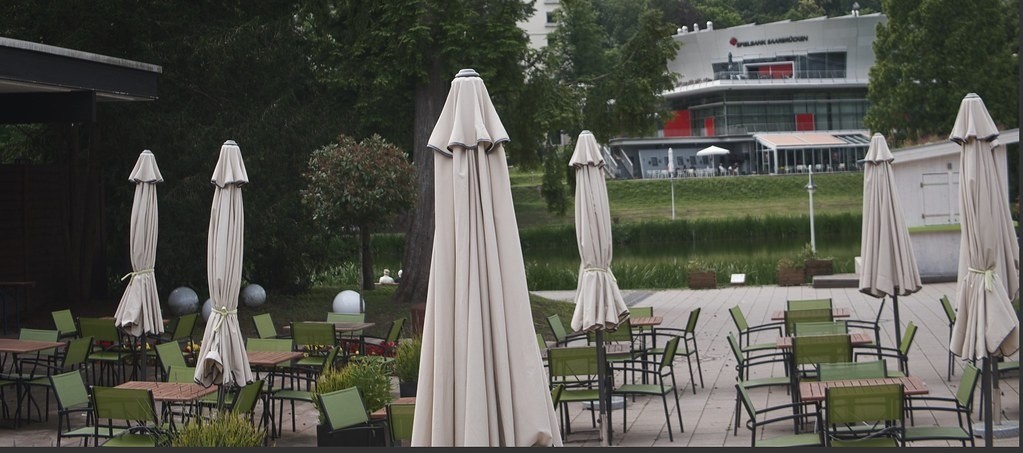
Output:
[774,257,806,286]
[687,259,717,289]
[799,243,833,275]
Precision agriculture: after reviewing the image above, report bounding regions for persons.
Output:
[732,163,738,169]
[379,269,394,283]
[394,270,403,283]
[719,163,727,172]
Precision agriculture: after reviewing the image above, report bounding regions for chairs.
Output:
[979,357,1020,420]
[797,163,847,173]
[645,166,740,177]
[785,309,834,395]
[891,360,981,448]
[536,333,571,440]
[851,320,918,427]
[729,305,788,380]
[587,318,647,402]
[836,298,886,361]
[0,308,415,447]
[545,347,607,445]
[940,294,956,381]
[784,298,833,311]
[791,334,853,434]
[726,331,790,427]
[607,336,686,442]
[547,313,596,428]
[824,382,906,448]
[735,382,832,448]
[653,307,704,394]
[607,306,657,385]
[814,359,890,447]
[793,320,848,337]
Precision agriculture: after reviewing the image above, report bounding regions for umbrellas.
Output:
[193,139,254,414]
[859,132,923,372]
[113,149,166,382]
[948,93,1019,447]
[410,69,563,448]
[567,129,631,446]
[697,145,729,168]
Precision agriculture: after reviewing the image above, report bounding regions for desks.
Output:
[798,376,928,448]
[777,332,872,345]
[100,317,171,381]
[282,321,375,356]
[0,339,66,427]
[244,351,303,447]
[771,307,850,321]
[112,382,217,434]
[370,397,416,447]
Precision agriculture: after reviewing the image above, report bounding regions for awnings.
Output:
[755,134,847,174]
